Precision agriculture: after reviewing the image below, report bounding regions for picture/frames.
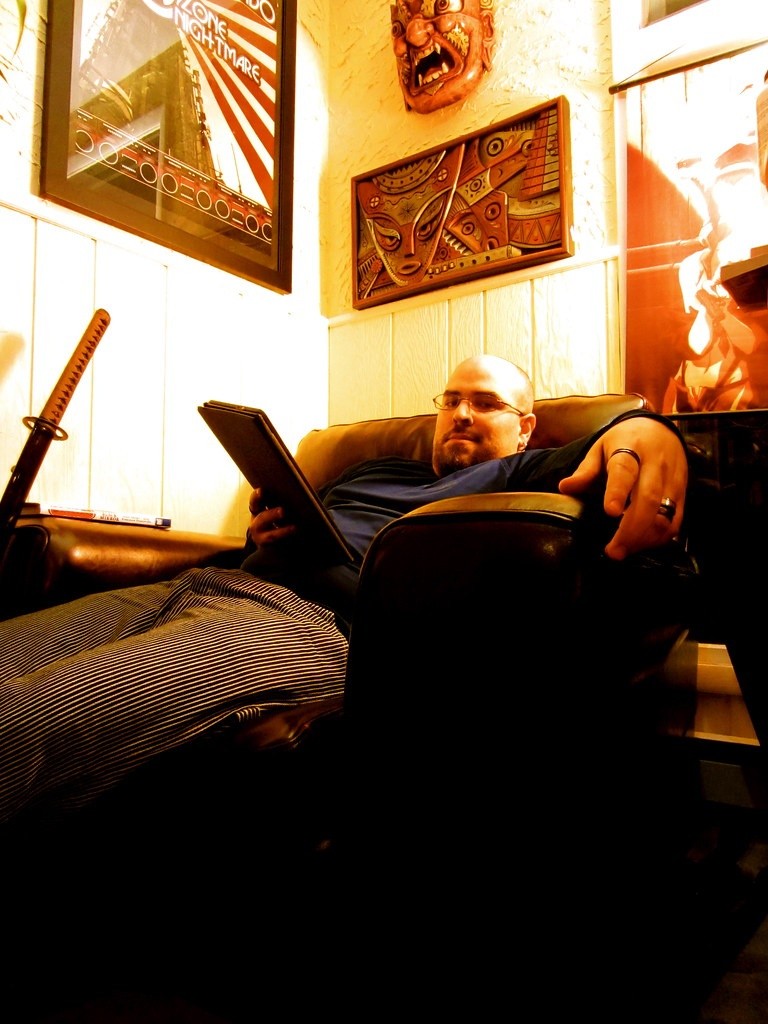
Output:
[40,0,299,303]
[347,93,580,313]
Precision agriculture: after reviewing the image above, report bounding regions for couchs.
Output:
[0,392,711,1024]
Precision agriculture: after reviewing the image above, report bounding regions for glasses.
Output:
[433,392,527,417]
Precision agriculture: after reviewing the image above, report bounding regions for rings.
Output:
[610,449,641,464]
[658,498,676,522]
[248,506,257,515]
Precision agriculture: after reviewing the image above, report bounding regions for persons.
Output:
[0,353,709,836]
